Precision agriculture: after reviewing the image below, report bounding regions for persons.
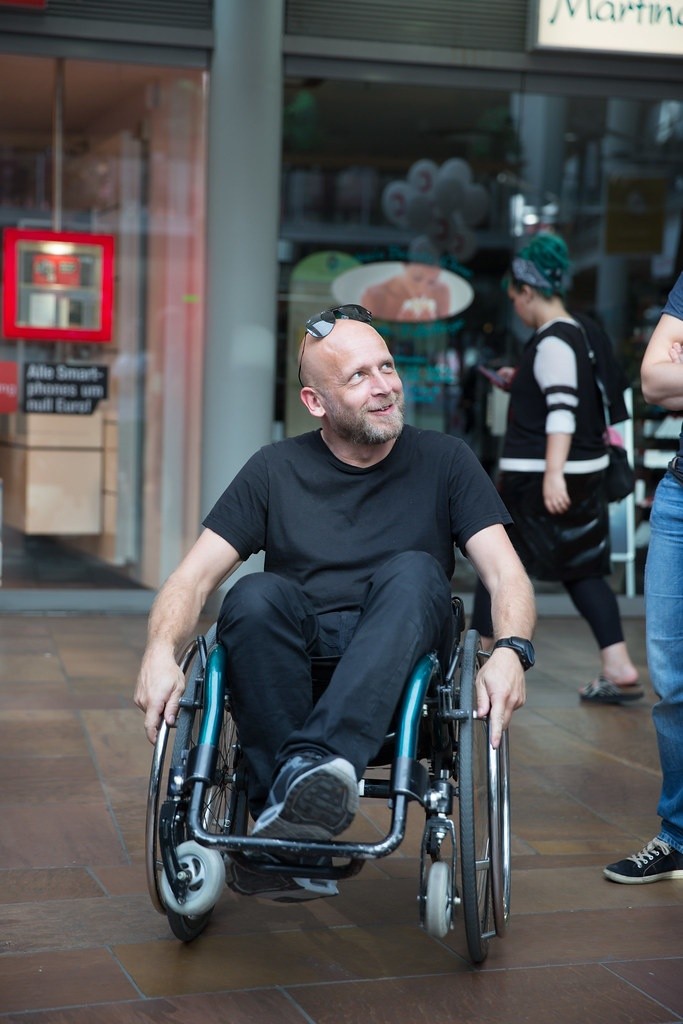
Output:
[464,229,646,702]
[602,268,682,885]
[132,317,538,906]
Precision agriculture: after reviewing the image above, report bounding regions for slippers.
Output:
[580,675,645,703]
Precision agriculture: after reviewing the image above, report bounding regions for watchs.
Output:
[490,636,537,672]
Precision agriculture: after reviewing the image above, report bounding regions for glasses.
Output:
[298,304,373,388]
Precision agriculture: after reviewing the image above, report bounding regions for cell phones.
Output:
[478,363,508,388]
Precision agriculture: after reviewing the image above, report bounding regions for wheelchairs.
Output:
[143,595,516,970]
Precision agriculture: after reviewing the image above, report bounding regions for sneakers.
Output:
[602,836,683,884]
[247,748,359,843]
[223,856,339,905]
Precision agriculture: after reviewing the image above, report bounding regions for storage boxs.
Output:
[57,256,80,285]
[32,255,57,283]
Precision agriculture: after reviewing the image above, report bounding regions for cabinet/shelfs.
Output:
[0,228,116,344]
[0,411,103,534]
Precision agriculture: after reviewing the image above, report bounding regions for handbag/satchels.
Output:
[600,426,635,505]
